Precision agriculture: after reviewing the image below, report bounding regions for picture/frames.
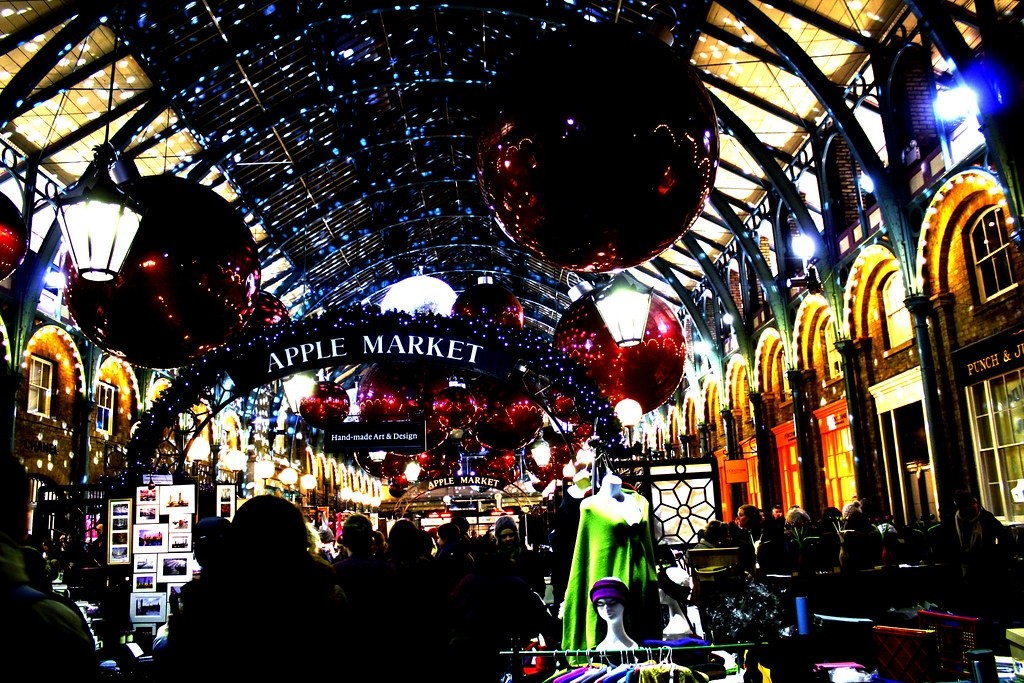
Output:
[133,624,157,635]
[136,505,159,524]
[108,498,132,565]
[133,524,168,553]
[130,593,166,623]
[133,574,157,592]
[157,553,193,583]
[134,554,157,573]
[137,486,159,505]
[160,484,196,515]
[167,582,186,602]
[169,513,191,533]
[169,533,191,552]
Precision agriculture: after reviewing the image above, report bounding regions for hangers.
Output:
[544,646,676,683]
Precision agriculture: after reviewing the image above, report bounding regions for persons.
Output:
[590,576,646,651]
[561,472,658,668]
[0,449,97,683]
[693,505,786,574]
[154,495,394,683]
[384,516,549,683]
[657,566,704,641]
[792,491,1024,663]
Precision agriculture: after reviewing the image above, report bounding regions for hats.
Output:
[591,577,629,611]
[495,517,517,536]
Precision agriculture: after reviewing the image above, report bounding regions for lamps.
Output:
[52,10,144,283]
[590,271,655,348]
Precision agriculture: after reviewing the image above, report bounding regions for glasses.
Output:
[499,532,517,539]
